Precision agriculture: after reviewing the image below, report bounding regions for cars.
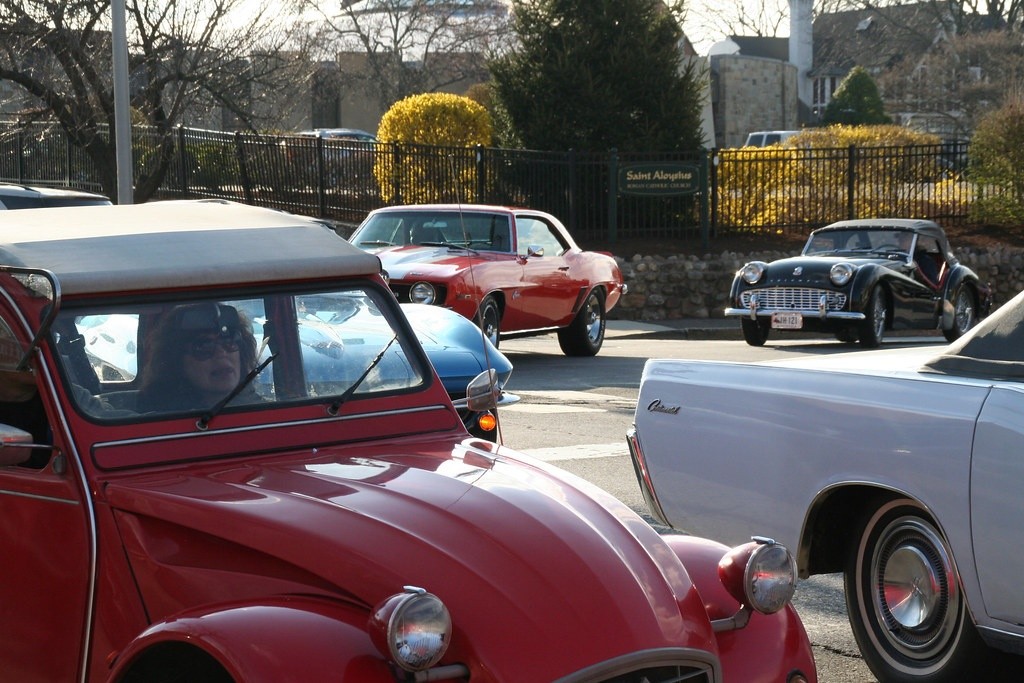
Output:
[348,202,628,358]
[0,194,817,683]
[0,184,110,217]
[627,288,1024,683]
[77,286,520,446]
[724,217,993,350]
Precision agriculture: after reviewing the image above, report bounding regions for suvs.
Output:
[279,128,377,189]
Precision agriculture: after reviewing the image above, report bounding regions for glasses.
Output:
[181,329,246,360]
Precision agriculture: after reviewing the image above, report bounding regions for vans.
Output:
[746,131,822,151]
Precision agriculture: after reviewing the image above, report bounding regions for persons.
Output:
[409,221,435,244]
[895,231,927,255]
[0,326,158,470]
[135,304,264,415]
